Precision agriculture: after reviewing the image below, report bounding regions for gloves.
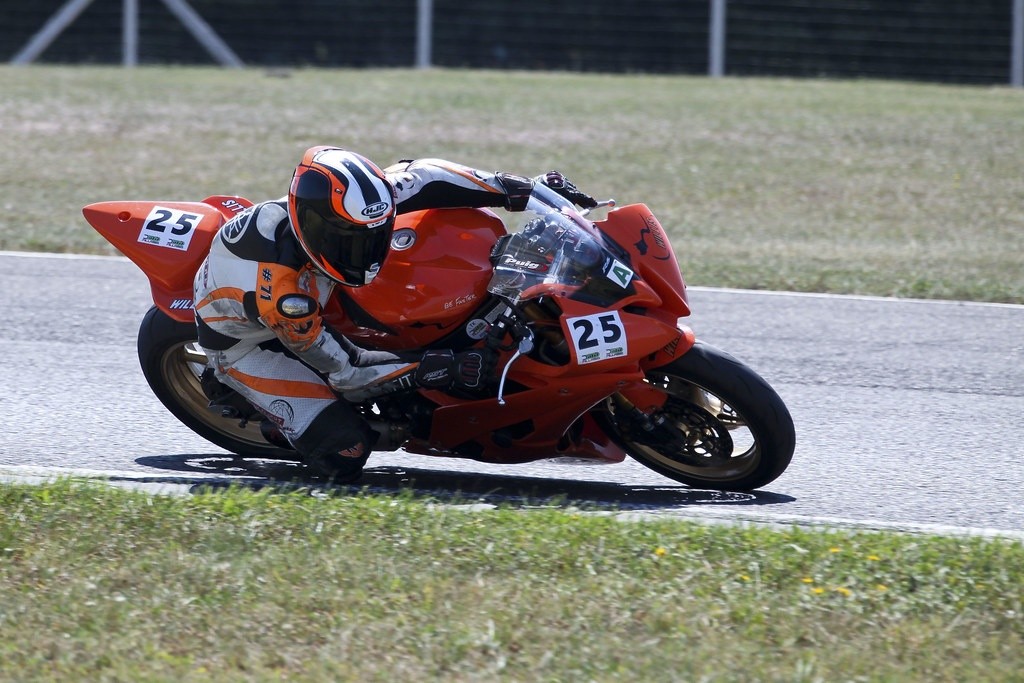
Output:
[415,348,500,398]
[495,170,598,213]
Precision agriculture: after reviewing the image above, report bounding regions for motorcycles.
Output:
[81,172,798,492]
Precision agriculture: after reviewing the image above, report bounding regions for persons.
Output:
[192,145,597,485]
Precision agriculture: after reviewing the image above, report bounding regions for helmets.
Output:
[287,145,396,287]
